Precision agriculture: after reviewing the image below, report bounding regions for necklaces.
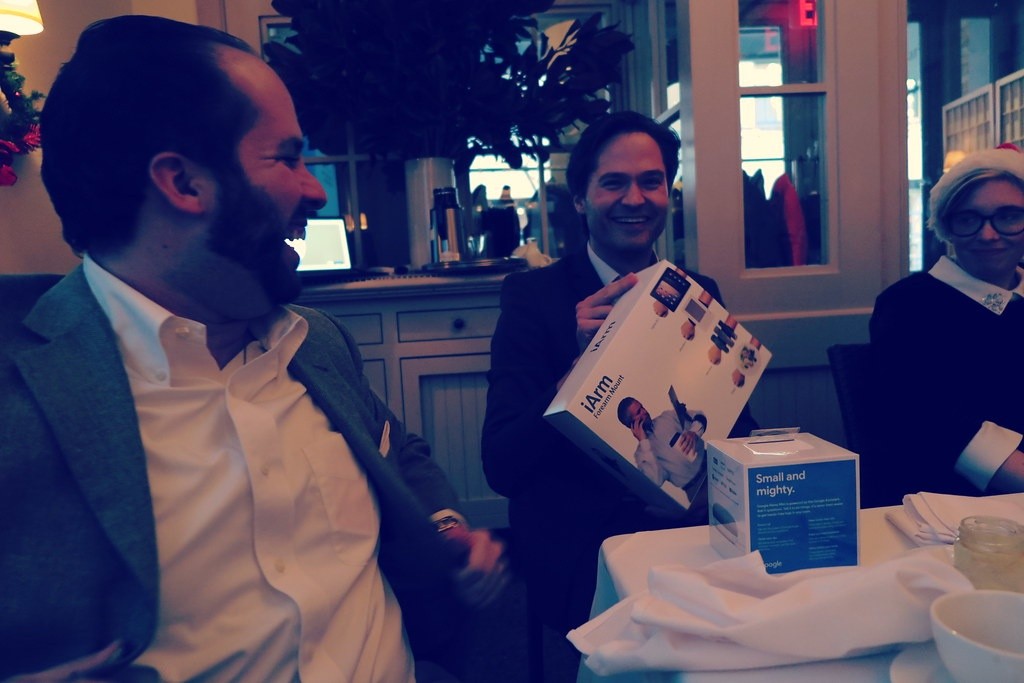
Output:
[243,330,250,366]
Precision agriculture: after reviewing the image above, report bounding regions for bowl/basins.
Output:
[931,590,1024,683]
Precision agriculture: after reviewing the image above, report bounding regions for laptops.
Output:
[284,217,390,285]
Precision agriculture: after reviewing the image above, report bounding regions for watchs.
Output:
[432,515,460,531]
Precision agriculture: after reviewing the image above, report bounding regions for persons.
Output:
[469,175,682,267]
[617,397,707,504]
[479,109,762,683]
[0,14,514,683]
[864,153,1024,510]
[654,269,762,387]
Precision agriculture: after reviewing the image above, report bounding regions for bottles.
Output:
[428,188,464,263]
[954,515,1024,593]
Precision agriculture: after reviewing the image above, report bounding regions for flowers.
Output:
[0,32,47,186]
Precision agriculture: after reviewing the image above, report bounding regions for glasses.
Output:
[940,204,1024,237]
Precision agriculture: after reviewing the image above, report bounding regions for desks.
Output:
[577,493,1024,683]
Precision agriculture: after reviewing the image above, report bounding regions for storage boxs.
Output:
[706,428,859,575]
[545,256,771,517]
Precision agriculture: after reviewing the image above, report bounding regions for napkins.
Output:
[884,488,1018,548]
[565,551,974,676]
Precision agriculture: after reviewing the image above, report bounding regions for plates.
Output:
[889,644,962,683]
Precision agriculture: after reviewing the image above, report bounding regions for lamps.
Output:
[0,0,44,34]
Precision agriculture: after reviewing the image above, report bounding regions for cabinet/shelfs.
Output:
[292,288,506,537]
